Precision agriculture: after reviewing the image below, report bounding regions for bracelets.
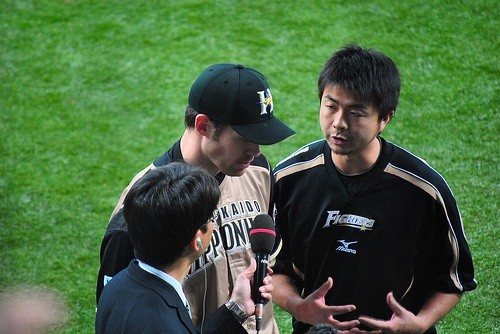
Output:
[225,300,248,324]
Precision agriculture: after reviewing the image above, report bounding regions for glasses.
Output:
[199,207,220,230]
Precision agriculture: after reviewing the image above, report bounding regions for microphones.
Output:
[250,214,277,320]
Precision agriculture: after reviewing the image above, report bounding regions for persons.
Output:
[274,47,476,334]
[96,64,295,334]
[95,164,274,334]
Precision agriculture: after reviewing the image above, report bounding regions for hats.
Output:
[188,64,297,145]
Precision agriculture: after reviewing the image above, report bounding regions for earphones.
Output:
[197,236,203,249]
